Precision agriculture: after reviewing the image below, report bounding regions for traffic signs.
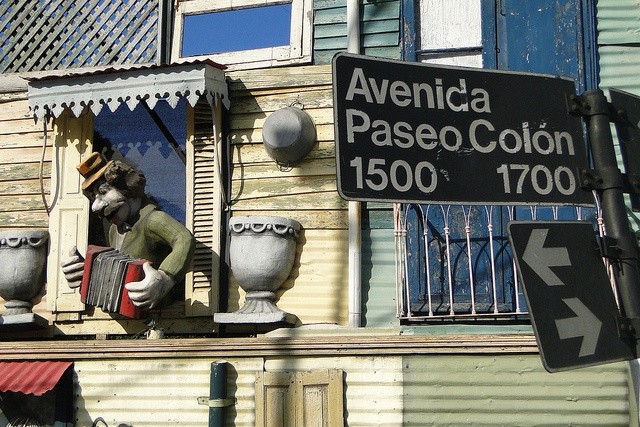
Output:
[332,52,597,203]
